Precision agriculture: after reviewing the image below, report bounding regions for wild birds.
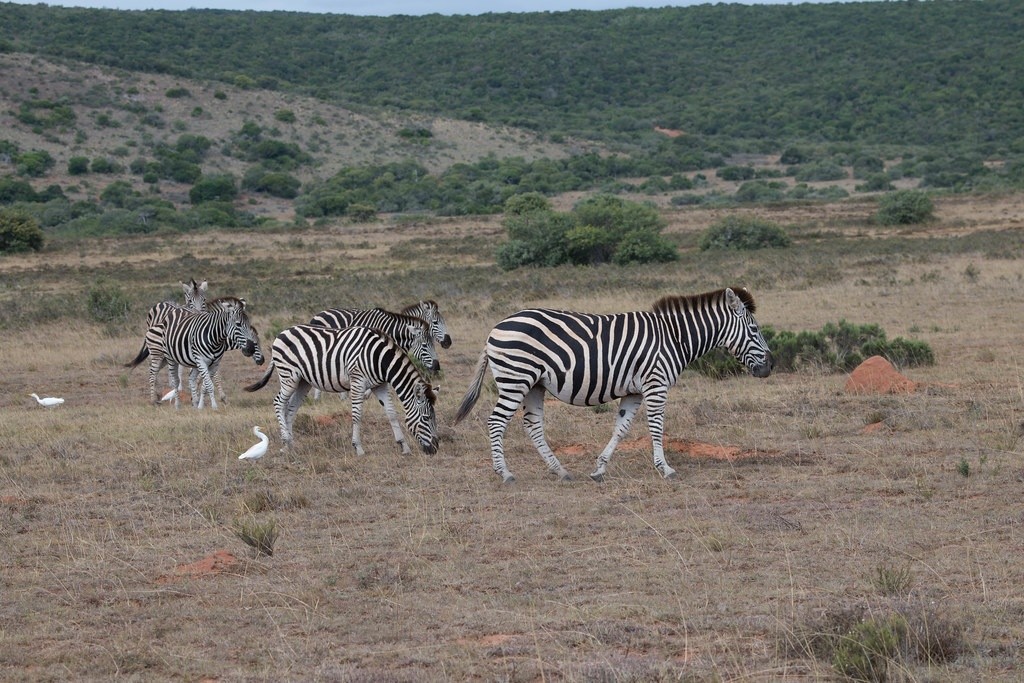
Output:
[28,393,65,409]
[238,426,269,463]
[161,379,183,407]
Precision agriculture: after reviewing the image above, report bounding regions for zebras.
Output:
[122,276,265,411]
[452,285,775,484]
[243,300,452,459]
